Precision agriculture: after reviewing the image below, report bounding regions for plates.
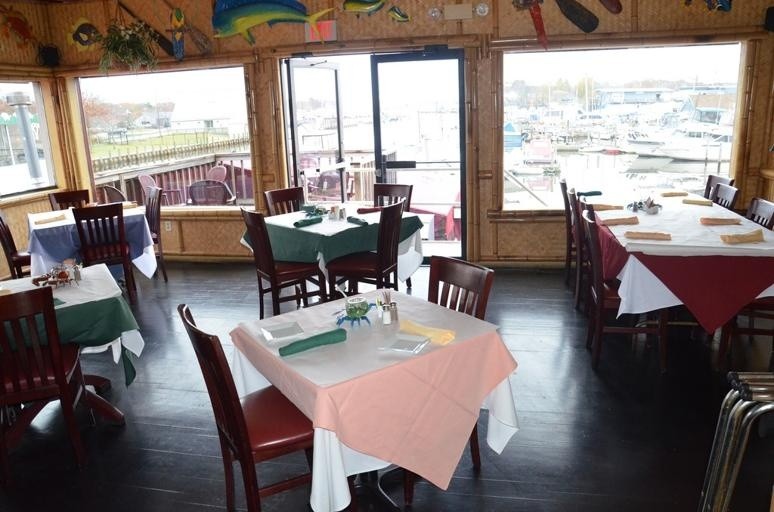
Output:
[375,330,431,357]
[259,320,307,345]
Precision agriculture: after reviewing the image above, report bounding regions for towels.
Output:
[600,215,638,226]
[0,286,18,299]
[623,230,672,242]
[346,213,368,228]
[357,205,382,214]
[590,203,625,212]
[576,190,604,196]
[718,227,766,245]
[32,212,70,225]
[293,215,324,228]
[699,216,743,226]
[662,191,690,196]
[399,318,457,347]
[682,197,715,206]
[277,327,348,358]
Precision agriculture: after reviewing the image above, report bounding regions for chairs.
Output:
[0,286,95,490]
[325,196,407,301]
[0,214,31,279]
[710,183,740,210]
[205,166,227,181]
[178,304,359,512]
[400,256,495,505]
[566,187,592,309]
[560,179,577,285]
[240,208,327,320]
[580,194,639,342]
[354,183,413,289]
[49,190,90,211]
[581,210,670,377]
[187,180,236,206]
[123,186,168,283]
[704,175,735,198]
[706,197,774,340]
[138,174,184,206]
[263,187,328,309]
[104,185,127,203]
[725,297,774,358]
[72,203,137,305]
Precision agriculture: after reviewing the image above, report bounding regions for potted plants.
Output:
[91,20,160,74]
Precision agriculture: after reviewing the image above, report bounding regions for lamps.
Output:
[475,3,486,18]
[427,8,441,21]
